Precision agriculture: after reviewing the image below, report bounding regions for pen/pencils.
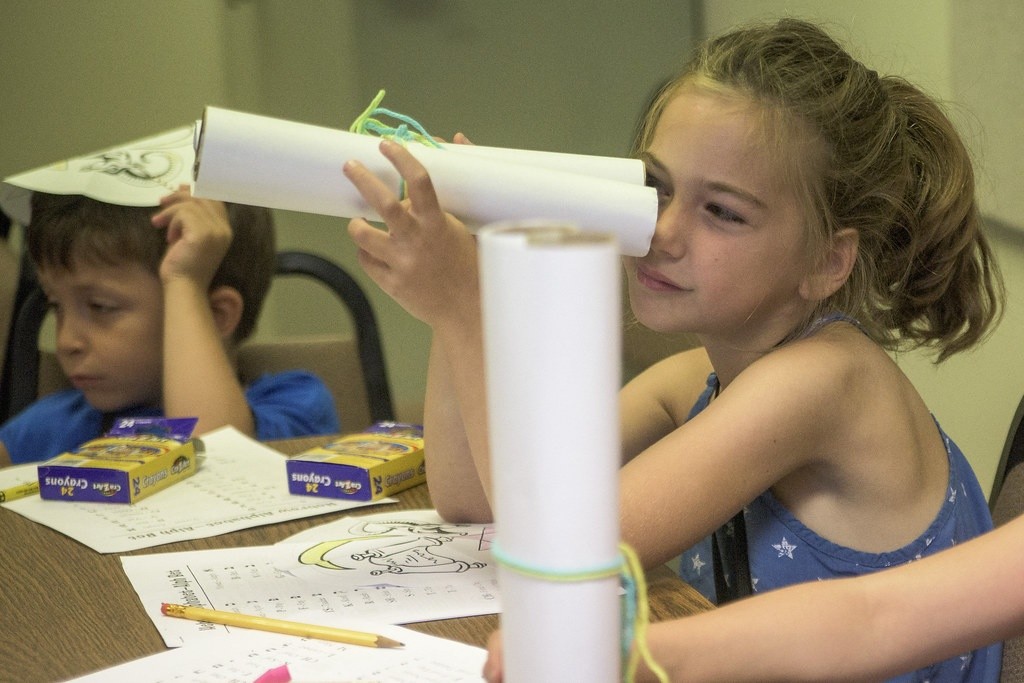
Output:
[161,603,405,648]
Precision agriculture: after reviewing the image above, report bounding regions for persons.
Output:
[342,17,1004,683]
[481,510,1024,683]
[0,191,341,471]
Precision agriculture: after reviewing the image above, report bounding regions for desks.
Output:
[0,434,719,683]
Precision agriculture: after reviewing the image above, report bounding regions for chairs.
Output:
[0,251,393,427]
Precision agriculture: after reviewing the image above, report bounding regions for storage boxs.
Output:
[38,417,202,505]
[287,421,426,501]
